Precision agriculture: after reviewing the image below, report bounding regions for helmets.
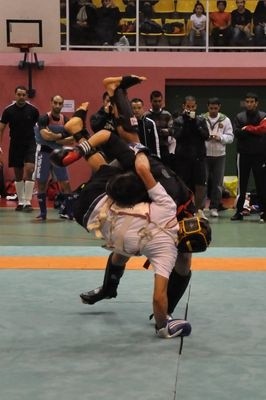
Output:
[175,215,212,254]
[106,173,149,208]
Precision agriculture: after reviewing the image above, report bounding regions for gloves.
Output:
[123,141,149,157]
[156,320,193,339]
[49,140,89,167]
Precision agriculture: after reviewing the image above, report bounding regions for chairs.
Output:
[117,14,255,52]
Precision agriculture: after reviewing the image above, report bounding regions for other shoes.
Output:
[58,210,70,220]
[218,204,229,212]
[23,202,34,213]
[209,208,220,218]
[196,210,208,220]
[259,210,266,222]
[34,214,47,221]
[15,203,25,212]
[230,211,244,221]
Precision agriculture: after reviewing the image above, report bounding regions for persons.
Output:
[59,0,266,52]
[0,75,266,338]
